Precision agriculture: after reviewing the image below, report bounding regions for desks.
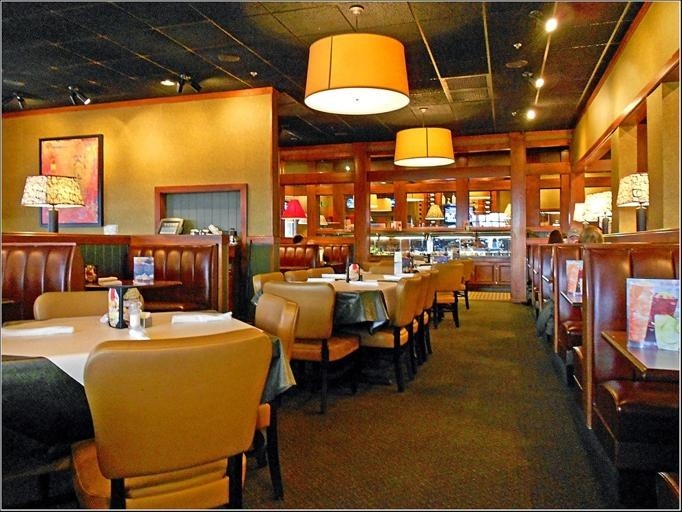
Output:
[85,278,183,293]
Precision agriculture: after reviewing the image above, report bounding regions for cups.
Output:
[566,228,580,245]
[627,282,680,350]
[568,263,585,293]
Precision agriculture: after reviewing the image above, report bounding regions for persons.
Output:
[564,228,581,244]
[293,236,327,267]
[535,225,605,337]
[548,230,563,243]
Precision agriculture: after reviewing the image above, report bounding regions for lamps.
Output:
[19,173,84,232]
[301,3,412,116]
[570,173,652,236]
[282,198,307,239]
[14,91,30,111]
[424,204,444,226]
[67,90,91,106]
[508,10,560,124]
[392,106,455,168]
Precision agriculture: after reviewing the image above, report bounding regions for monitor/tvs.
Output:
[444,205,474,224]
[346,198,354,209]
[156,217,185,234]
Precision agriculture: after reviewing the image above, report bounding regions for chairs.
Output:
[252,239,475,415]
[1,290,300,509]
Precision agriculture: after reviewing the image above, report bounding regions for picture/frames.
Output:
[36,134,106,227]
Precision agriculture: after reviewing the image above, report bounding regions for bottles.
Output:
[443,246,449,260]
[405,253,434,273]
[85,265,94,283]
[127,298,141,329]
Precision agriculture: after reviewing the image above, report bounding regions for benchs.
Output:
[2,242,86,320]
[524,236,680,510]
[110,238,229,312]
